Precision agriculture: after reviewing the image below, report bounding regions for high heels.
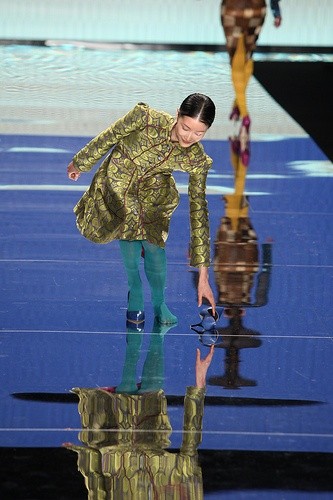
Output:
[189,305,224,332]
[242,115,252,133]
[227,105,240,120]
[126,290,146,325]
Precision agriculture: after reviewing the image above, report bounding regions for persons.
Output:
[62,344,215,500]
[65,93,217,326]
[187,132,273,319]
[221,0,281,134]
